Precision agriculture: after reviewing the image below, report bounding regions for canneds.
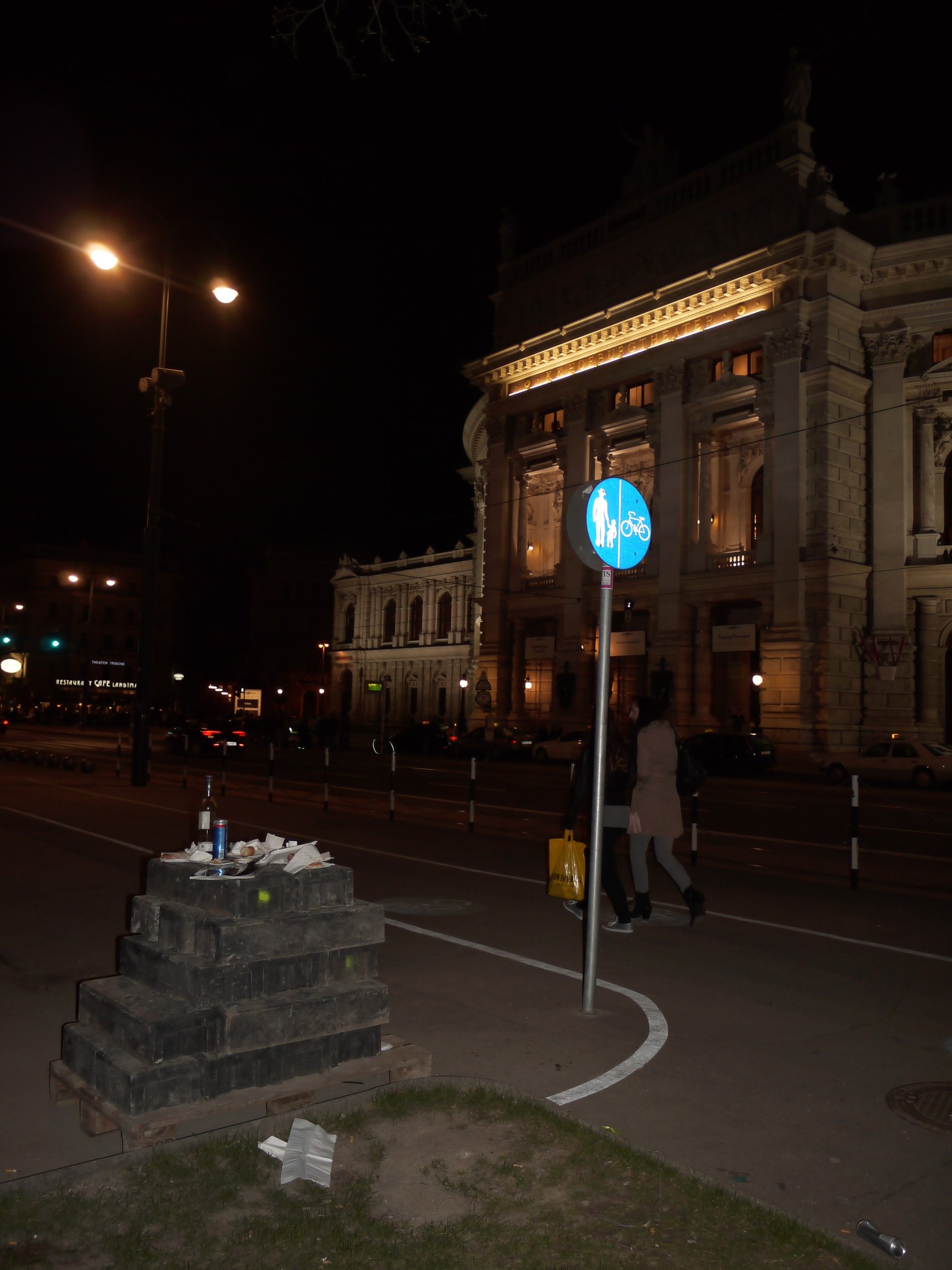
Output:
[212,819,228,860]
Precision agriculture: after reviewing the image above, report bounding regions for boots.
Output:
[629,892,652,920]
[681,886,706,927]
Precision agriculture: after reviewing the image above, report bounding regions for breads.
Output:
[240,846,254,856]
[307,862,323,868]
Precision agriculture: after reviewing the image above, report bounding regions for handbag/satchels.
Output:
[549,829,587,900]
[670,724,702,794]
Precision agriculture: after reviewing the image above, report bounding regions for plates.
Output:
[302,863,334,870]
[201,859,235,866]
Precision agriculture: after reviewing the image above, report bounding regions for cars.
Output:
[167,719,246,758]
[383,720,952,788]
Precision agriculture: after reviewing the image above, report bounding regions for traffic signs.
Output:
[586,478,651,569]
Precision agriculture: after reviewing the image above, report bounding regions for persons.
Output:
[627,694,706,925]
[561,707,635,934]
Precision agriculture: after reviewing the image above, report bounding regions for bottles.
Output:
[198,775,218,856]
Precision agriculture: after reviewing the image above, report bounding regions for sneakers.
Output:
[602,920,633,933]
[563,900,583,920]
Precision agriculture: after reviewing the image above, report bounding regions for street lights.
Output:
[81,240,239,784]
[68,575,116,725]
